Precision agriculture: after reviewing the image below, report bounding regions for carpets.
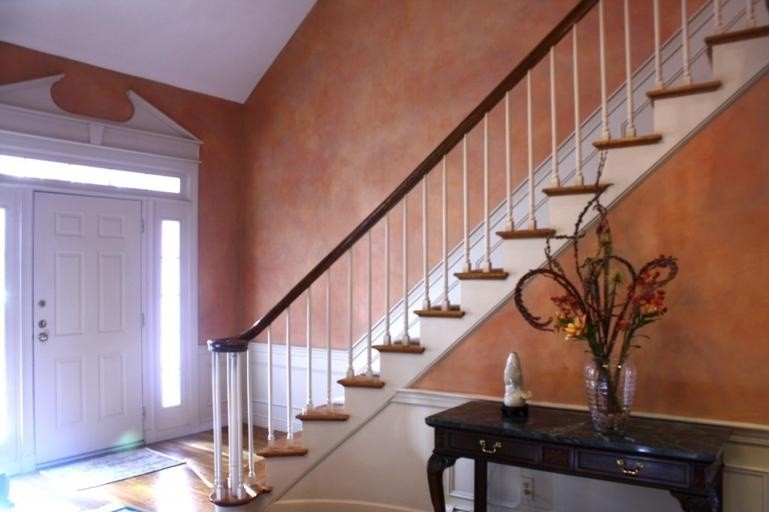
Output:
[40,446,186,492]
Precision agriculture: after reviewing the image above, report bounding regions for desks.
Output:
[425,399,732,512]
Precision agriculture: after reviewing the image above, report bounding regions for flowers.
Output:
[516,187,676,345]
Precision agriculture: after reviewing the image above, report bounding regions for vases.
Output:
[584,357,635,437]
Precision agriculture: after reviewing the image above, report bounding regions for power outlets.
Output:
[519,476,533,501]
